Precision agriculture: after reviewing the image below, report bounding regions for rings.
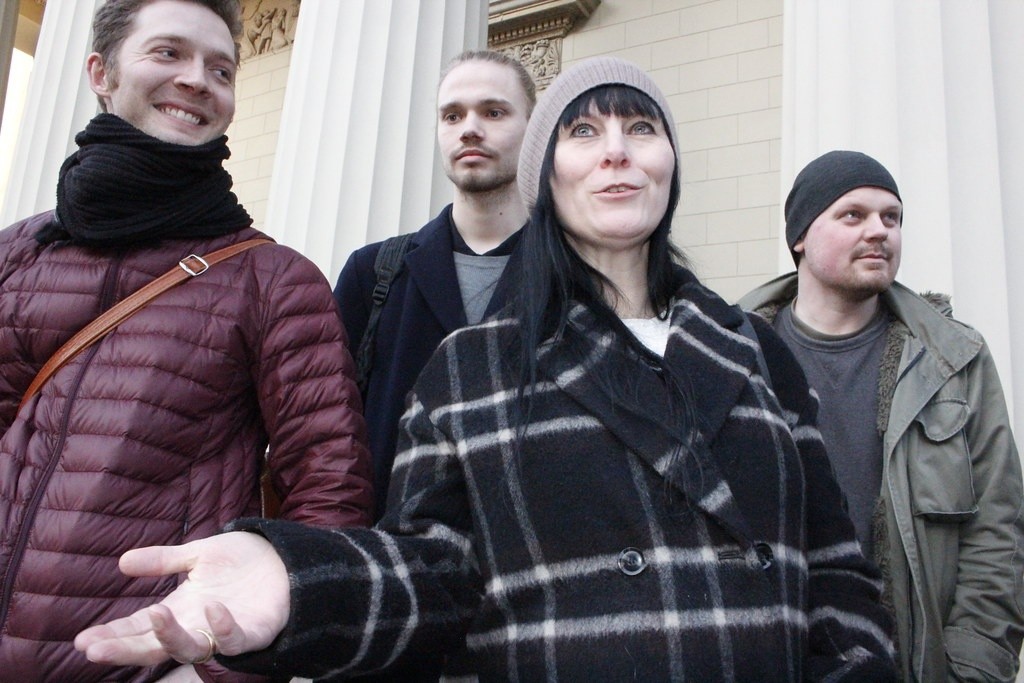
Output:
[181,627,216,665]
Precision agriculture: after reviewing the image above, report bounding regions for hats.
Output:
[785,150,904,266]
[516,57,683,220]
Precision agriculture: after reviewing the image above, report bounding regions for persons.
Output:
[73,53,905,682]
[330,47,536,516]
[1,0,373,683]
[735,150,1024,681]
